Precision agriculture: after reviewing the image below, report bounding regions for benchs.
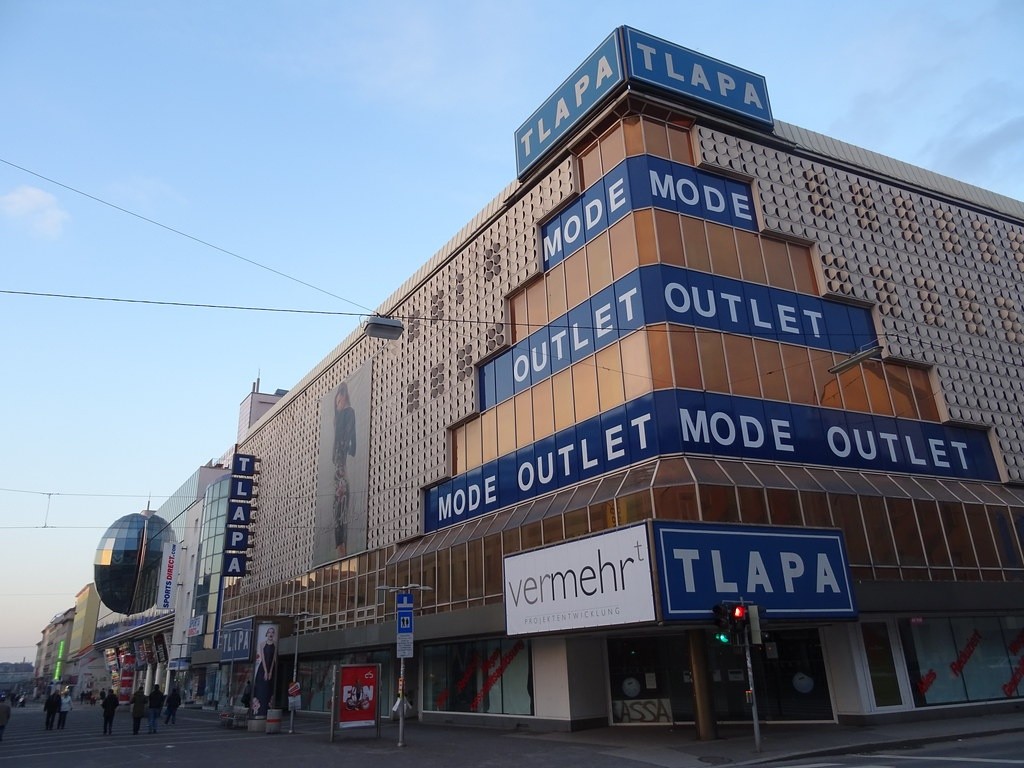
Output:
[217,705,248,728]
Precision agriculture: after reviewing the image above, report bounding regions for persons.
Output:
[101,689,119,735]
[165,688,181,724]
[0,697,10,742]
[80,688,106,704]
[128,686,146,735]
[241,680,251,708]
[254,628,275,715]
[0,686,26,709]
[44,690,73,730]
[148,684,165,734]
[330,381,358,559]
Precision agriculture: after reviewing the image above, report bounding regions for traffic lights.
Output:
[712,603,745,647]
